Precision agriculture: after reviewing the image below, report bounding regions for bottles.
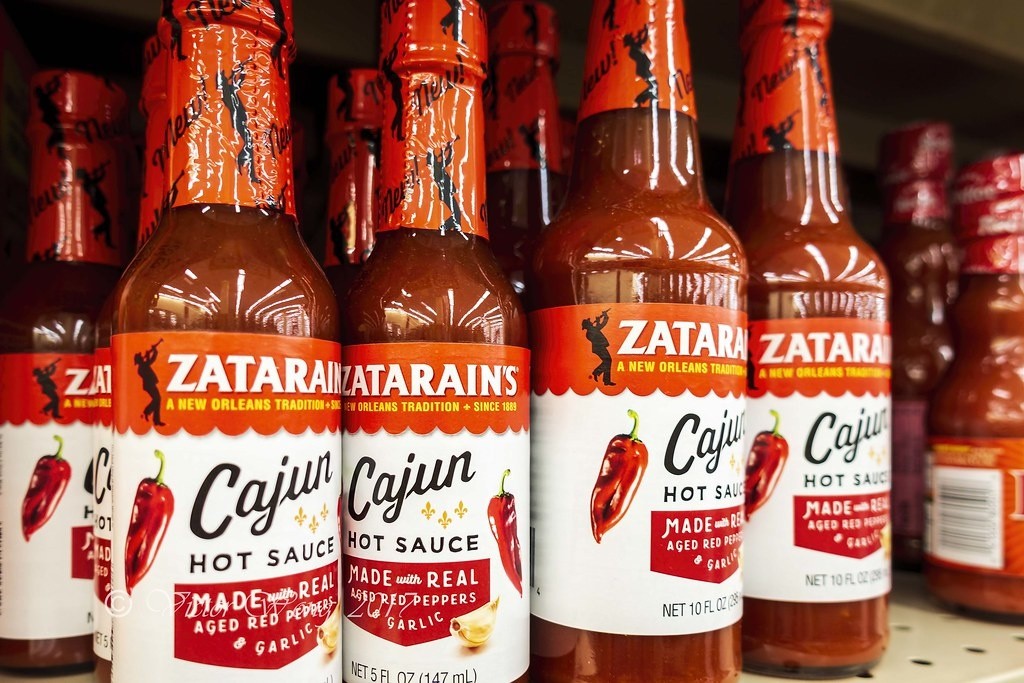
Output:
[0,0,1024,683]
